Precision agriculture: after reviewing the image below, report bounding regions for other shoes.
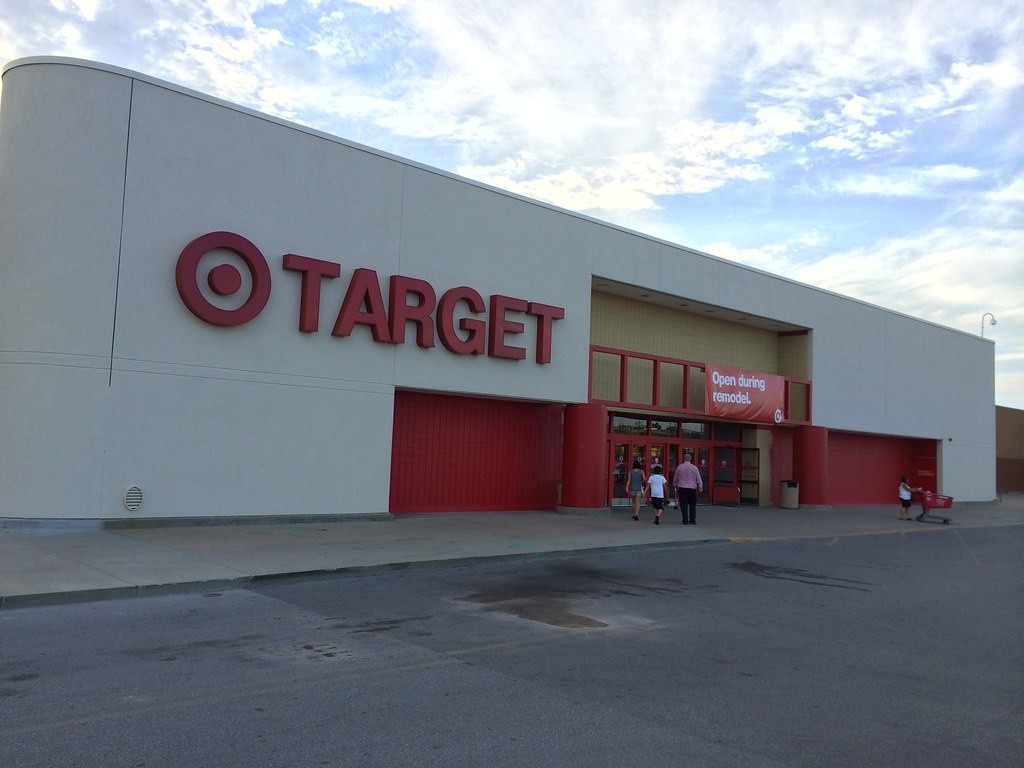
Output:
[690,519,695,525]
[655,516,659,525]
[907,517,912,520]
[683,520,687,525]
[633,515,638,521]
[899,518,906,521]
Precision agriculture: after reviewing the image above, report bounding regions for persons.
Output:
[643,466,668,525]
[672,453,704,525]
[897,475,921,521]
[626,460,646,521]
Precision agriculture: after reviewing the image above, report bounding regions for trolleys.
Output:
[916,487,954,526]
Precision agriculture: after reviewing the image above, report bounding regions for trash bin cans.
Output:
[779,480,799,509]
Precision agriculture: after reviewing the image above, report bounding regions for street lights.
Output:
[981,313,997,339]
